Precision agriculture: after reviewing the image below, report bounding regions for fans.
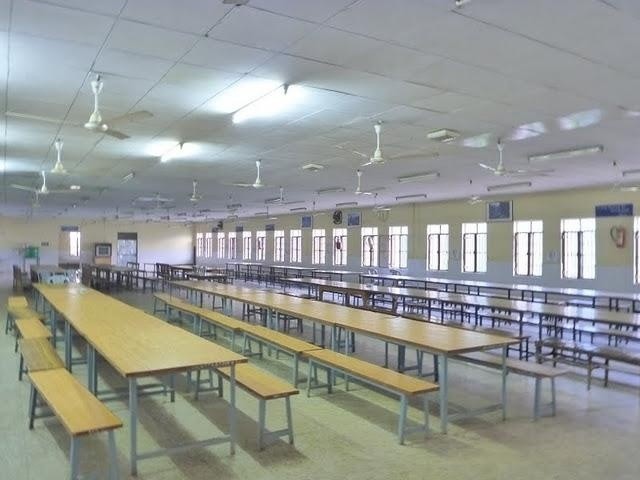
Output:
[475,140,530,178]
[332,122,439,197]
[224,156,280,190]
[3,69,153,200]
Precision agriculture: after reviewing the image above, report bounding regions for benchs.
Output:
[153,290,437,451]
[5,289,123,480]
[5,270,302,292]
[249,301,565,424]
[303,269,639,397]
[183,335,295,453]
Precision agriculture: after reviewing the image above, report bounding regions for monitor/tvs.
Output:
[301,215,312,229]
[347,213,362,226]
[486,200,513,223]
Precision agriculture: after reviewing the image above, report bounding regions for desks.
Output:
[173,281,522,435]
[32,279,244,477]
[223,263,314,294]
